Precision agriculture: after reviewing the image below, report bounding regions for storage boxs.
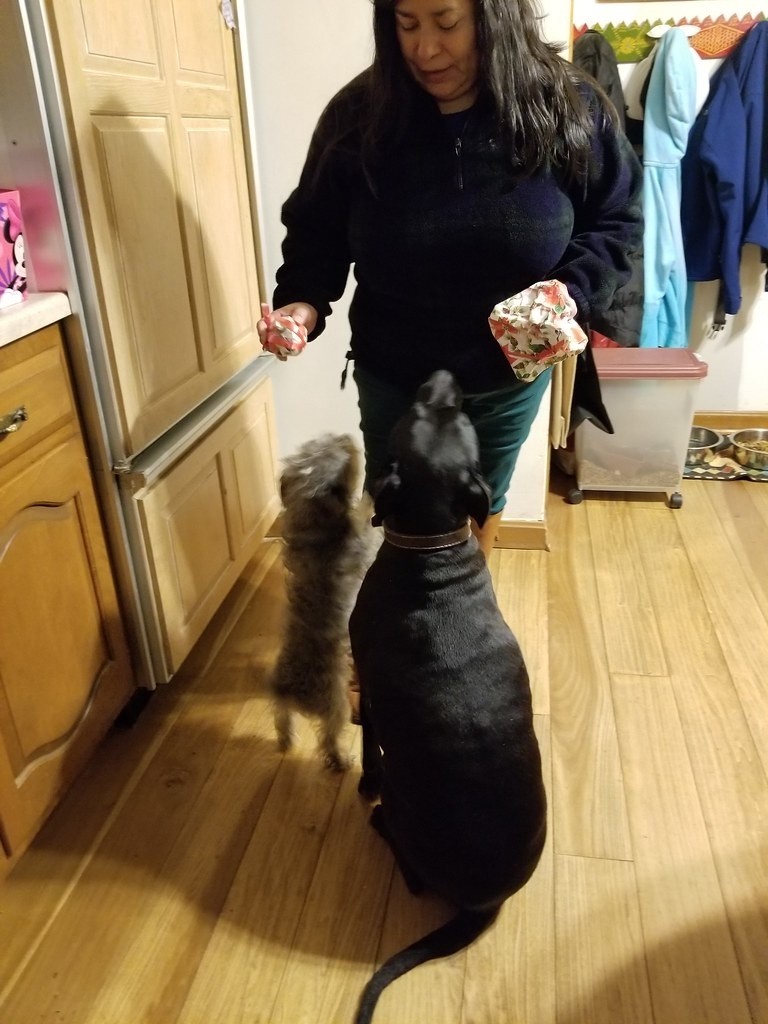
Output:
[567,348,708,509]
[0,188,28,309]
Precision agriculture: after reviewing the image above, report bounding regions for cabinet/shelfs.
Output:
[28,1,268,476]
[0,323,138,874]
[121,357,282,684]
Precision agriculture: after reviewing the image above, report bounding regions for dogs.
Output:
[348,364,548,1024]
[269,434,384,776]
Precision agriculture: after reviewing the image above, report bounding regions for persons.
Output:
[257,0,646,726]
